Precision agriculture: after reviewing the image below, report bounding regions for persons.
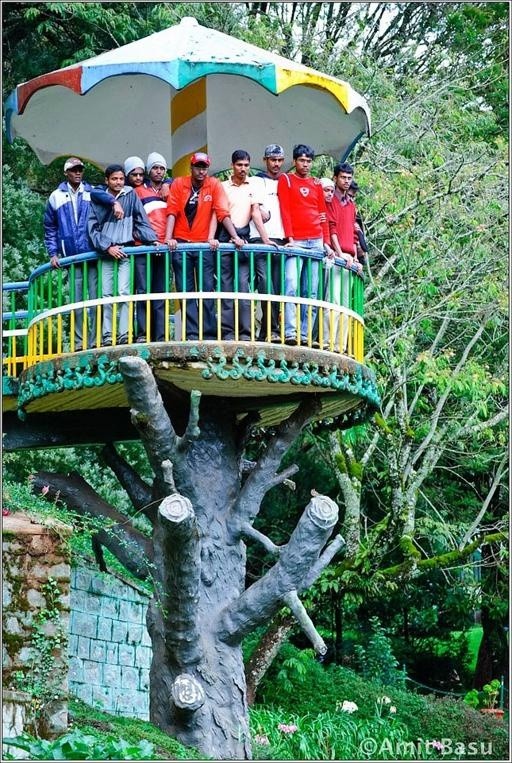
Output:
[320,178,335,203]
[43,157,99,351]
[88,165,162,346]
[208,150,280,341]
[249,144,283,344]
[321,163,356,354]
[90,156,174,343]
[346,182,365,276]
[277,144,335,346]
[133,152,167,343]
[164,152,244,340]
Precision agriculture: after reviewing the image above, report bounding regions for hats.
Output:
[264,144,284,158]
[124,156,145,176]
[146,152,167,175]
[321,177,335,188]
[64,157,84,172]
[191,153,210,166]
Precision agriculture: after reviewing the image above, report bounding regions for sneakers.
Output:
[93,342,103,347]
[136,336,145,342]
[324,344,330,350]
[342,352,354,358]
[285,337,297,344]
[120,335,128,344]
[103,337,112,345]
[312,341,319,348]
[75,344,82,351]
[301,341,307,346]
[271,333,282,343]
